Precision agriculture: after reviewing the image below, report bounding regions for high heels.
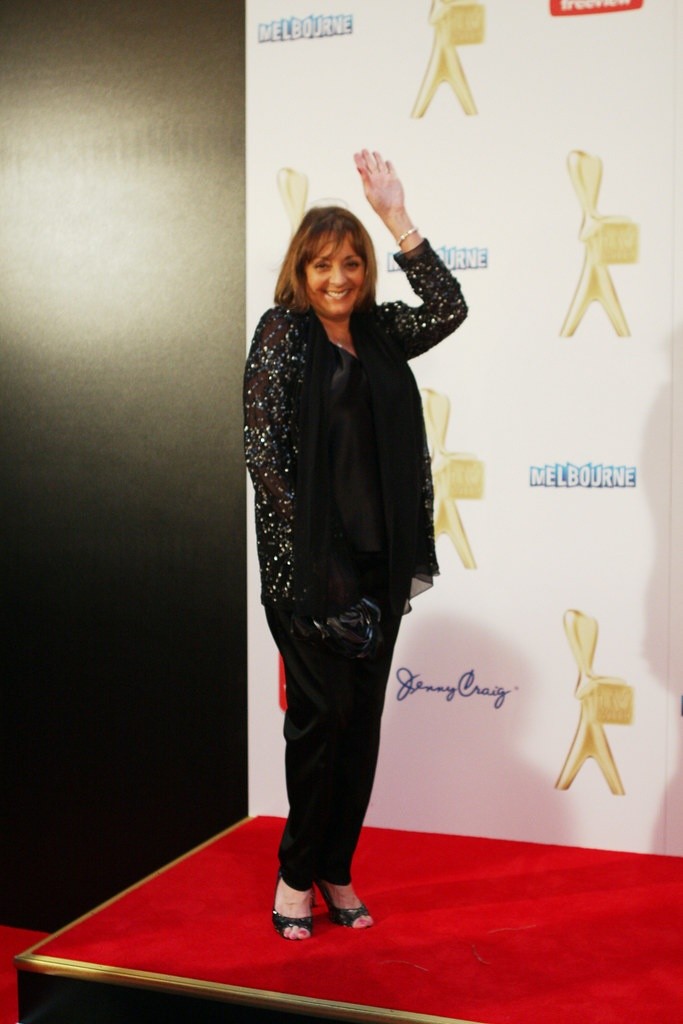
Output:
[311,880,372,928]
[272,867,313,940]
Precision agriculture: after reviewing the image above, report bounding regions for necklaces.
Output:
[336,342,342,348]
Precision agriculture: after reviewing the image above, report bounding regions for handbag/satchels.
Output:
[291,594,386,664]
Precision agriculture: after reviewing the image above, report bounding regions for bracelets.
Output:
[398,227,418,245]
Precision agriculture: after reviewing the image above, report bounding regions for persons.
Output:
[242,149,470,941]
